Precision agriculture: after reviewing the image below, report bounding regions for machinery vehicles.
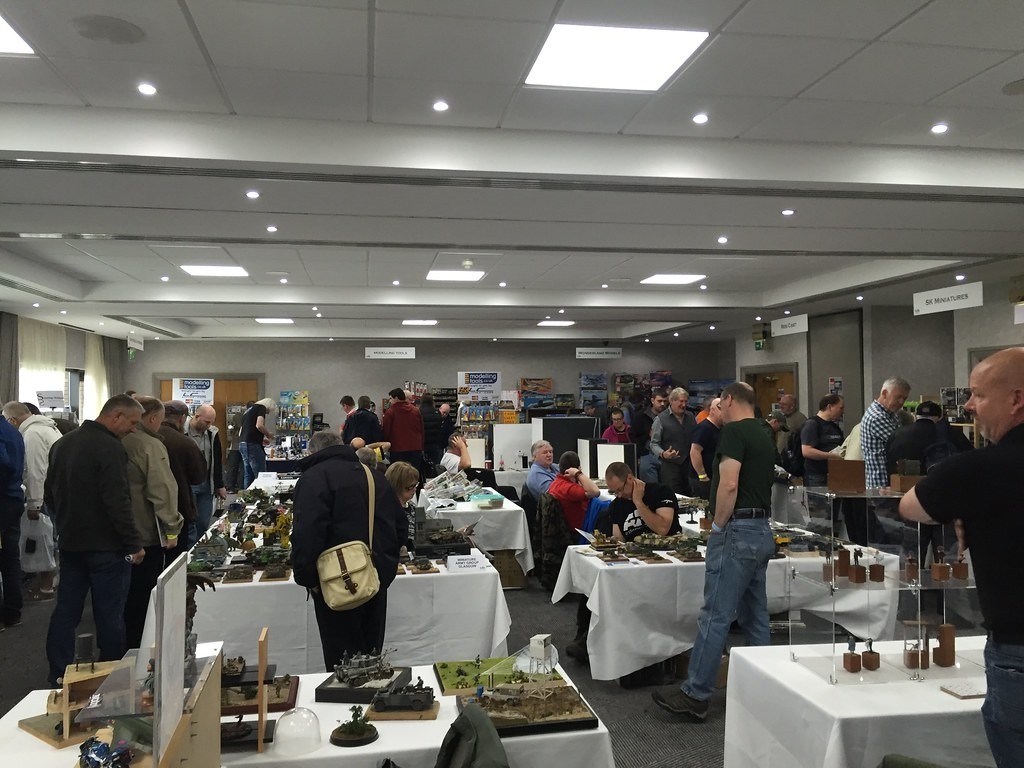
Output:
[334,647,398,686]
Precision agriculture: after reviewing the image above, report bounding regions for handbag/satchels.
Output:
[18,510,56,572]
[316,540,381,611]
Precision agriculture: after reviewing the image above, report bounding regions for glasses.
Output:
[608,477,627,495]
[716,395,734,410]
[405,482,419,490]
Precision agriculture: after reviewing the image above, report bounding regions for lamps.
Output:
[462,258,473,268]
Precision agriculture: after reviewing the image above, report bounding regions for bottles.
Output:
[404,380,427,396]
[516,451,528,468]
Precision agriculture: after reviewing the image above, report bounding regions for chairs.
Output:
[435,701,510,767]
[464,468,520,505]
[530,493,576,592]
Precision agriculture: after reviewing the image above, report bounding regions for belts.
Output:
[729,511,768,519]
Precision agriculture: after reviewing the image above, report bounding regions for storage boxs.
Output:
[579,369,609,414]
[457,372,575,406]
[499,409,519,425]
[489,551,520,587]
[890,474,927,491]
[412,537,471,559]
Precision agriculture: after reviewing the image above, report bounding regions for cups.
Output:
[484,460,492,470]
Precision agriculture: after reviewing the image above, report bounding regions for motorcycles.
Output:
[78,736,135,768]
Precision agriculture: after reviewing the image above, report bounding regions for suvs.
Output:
[371,684,436,712]
[481,683,526,706]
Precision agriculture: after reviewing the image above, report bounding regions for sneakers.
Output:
[651,689,708,723]
[24,587,57,602]
[566,628,587,655]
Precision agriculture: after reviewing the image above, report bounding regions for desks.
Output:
[0,406,996,768]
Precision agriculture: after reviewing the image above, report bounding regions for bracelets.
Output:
[167,535,178,539]
[575,471,582,477]
[699,473,707,479]
[712,521,722,531]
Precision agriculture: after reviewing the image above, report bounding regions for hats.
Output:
[164,399,194,420]
[916,401,941,418]
[768,410,790,432]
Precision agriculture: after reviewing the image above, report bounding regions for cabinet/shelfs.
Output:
[63,660,135,739]
[431,387,457,424]
[788,490,987,684]
[221,626,277,753]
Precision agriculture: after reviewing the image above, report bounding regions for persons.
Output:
[290,432,409,672]
[239,398,277,489]
[143,659,155,692]
[0,390,227,688]
[766,394,845,536]
[605,462,682,542]
[527,440,558,501]
[386,462,474,538]
[549,451,600,537]
[440,432,471,474]
[339,388,453,499]
[582,388,723,500]
[839,377,975,623]
[652,383,777,721]
[900,347,1024,768]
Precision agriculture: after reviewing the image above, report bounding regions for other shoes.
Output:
[0,623,6,632]
[229,489,234,494]
[10,619,22,627]
